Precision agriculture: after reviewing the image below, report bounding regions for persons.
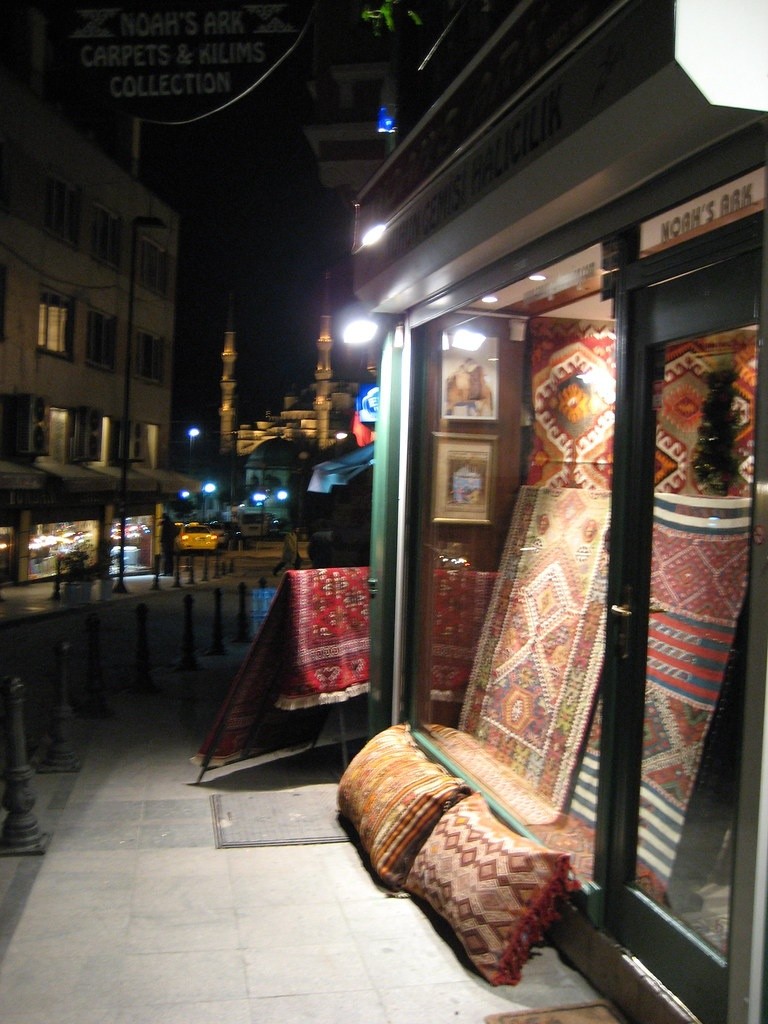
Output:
[159,511,176,576]
[271,526,303,576]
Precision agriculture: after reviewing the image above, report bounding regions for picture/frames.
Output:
[438,329,501,424]
[430,431,497,525]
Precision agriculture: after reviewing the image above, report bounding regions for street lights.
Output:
[110,215,170,594]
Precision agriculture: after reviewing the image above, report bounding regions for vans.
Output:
[238,510,269,537]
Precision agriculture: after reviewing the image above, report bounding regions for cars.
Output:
[173,522,217,555]
[209,523,231,546]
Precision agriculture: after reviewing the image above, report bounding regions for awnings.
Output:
[0,455,203,494]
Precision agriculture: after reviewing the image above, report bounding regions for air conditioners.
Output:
[16,393,50,455]
[73,405,102,460]
[119,419,145,459]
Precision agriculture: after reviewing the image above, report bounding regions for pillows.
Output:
[337,723,472,893]
[403,790,575,987]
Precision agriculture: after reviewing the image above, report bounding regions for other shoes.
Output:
[160,571,173,577]
[272,569,276,576]
